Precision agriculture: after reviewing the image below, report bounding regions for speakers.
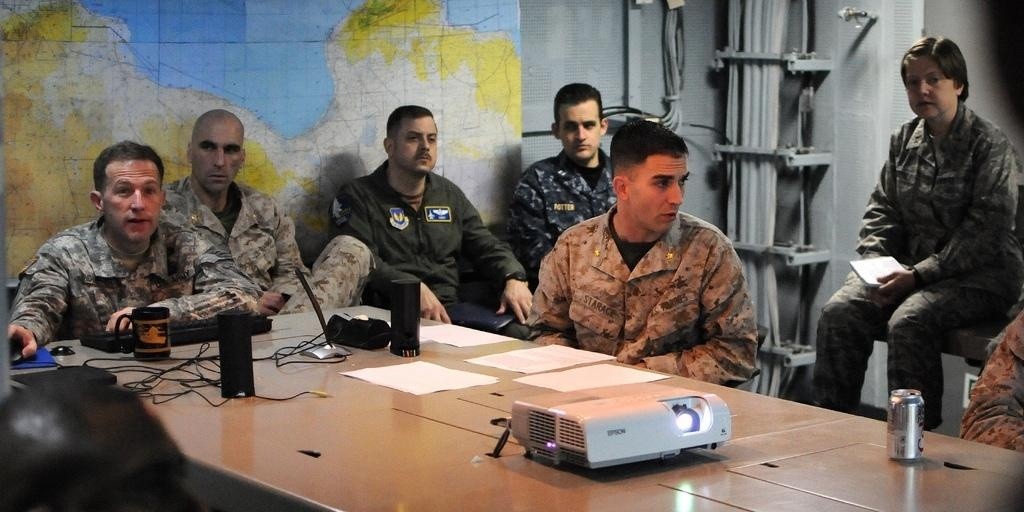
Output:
[390,278,423,357]
[214,306,254,398]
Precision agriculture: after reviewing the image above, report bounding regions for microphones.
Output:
[289,266,350,359]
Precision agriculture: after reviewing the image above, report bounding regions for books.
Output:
[848,254,907,288]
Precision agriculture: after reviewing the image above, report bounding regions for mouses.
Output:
[10,351,23,363]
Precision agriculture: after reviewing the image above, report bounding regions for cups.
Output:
[114,306,171,362]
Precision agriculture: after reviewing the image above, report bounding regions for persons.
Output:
[959,310,1024,454]
[160,109,378,318]
[8,140,261,362]
[502,83,619,293]
[526,115,759,386]
[329,104,534,340]
[810,33,1024,430]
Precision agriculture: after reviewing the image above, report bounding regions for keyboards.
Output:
[80,311,273,353]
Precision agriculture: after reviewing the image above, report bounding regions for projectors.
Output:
[510,384,732,470]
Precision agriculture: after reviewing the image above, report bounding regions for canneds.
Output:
[887,388,924,460]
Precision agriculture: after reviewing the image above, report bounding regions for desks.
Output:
[12,305,1024,512]
